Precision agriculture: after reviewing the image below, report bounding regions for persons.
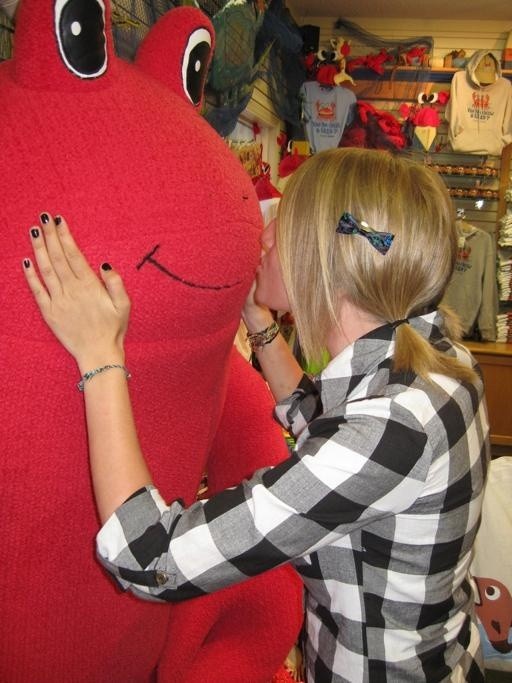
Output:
[19,147,492,683]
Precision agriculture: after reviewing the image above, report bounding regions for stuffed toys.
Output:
[0,0,306,683]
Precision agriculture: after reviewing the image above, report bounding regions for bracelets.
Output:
[247,321,280,350]
[76,364,132,392]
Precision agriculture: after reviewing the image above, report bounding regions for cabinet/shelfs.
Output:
[459,337,512,445]
